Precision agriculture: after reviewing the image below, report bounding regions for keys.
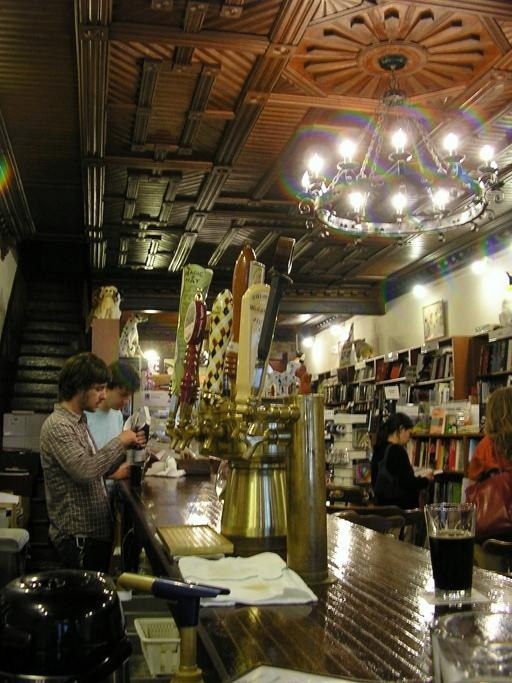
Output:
[78,546,85,569]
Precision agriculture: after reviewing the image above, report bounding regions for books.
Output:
[310,338,508,510]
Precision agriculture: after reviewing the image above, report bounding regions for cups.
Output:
[424,502,477,596]
[128,464,142,493]
[430,611,512,683]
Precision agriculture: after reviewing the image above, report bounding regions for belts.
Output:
[66,537,109,546]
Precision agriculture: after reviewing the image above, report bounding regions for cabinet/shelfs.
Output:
[300,331,509,533]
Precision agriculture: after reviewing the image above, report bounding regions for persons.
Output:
[468,387,512,578]
[39,352,147,574]
[83,361,141,505]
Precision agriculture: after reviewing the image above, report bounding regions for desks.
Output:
[116,470,512,682]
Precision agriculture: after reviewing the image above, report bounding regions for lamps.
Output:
[292,40,505,245]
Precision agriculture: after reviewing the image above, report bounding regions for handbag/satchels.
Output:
[373,444,401,499]
[465,472,512,540]
